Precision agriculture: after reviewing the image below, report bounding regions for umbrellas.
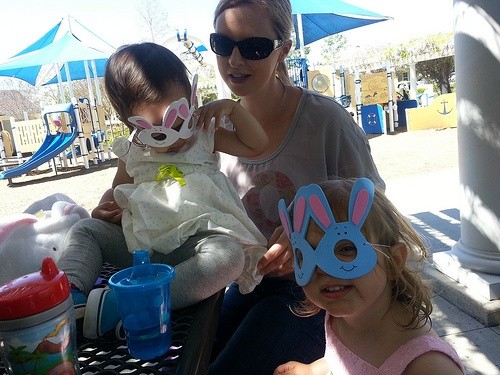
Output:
[287,0,395,89]
[0,16,119,169]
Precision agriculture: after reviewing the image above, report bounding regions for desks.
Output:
[0,263,227,375]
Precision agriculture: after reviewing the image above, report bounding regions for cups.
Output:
[108,249,176,361]
[0,256,82,375]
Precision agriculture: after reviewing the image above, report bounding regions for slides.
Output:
[66,131,105,158]
[1,130,78,182]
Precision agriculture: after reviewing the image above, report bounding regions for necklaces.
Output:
[233,76,287,112]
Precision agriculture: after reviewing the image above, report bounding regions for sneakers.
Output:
[83,285,122,340]
[69,287,87,320]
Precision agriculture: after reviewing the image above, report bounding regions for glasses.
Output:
[210,33,287,60]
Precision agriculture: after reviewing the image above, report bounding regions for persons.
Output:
[403,90,408,100]
[54,42,272,340]
[274,177,468,375]
[92,0,384,375]
